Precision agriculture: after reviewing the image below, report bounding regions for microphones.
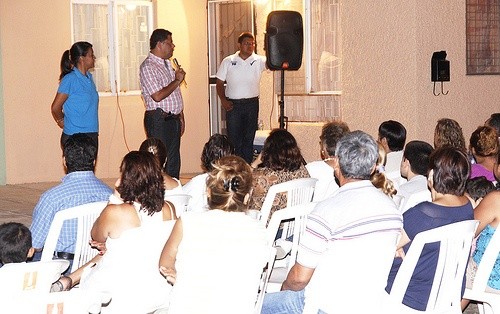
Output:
[173,58,187,87]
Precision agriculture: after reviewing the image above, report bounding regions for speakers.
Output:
[264,10,304,70]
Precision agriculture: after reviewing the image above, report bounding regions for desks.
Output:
[253,129,272,155]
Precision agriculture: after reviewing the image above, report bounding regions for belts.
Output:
[54,252,74,259]
[230,97,258,103]
[146,110,181,120]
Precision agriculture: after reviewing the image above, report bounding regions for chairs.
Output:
[388,219,481,314]
[243,177,320,240]
[249,241,278,314]
[0,259,71,314]
[40,199,110,288]
[254,201,318,292]
[462,223,500,314]
[403,189,432,214]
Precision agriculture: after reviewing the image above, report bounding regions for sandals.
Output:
[52,276,72,292]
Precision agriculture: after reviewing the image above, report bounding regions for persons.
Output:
[51,41,100,149]
[216,31,268,165]
[138,28,186,179]
[0,112,500,314]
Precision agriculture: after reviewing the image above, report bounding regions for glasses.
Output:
[241,42,256,47]
